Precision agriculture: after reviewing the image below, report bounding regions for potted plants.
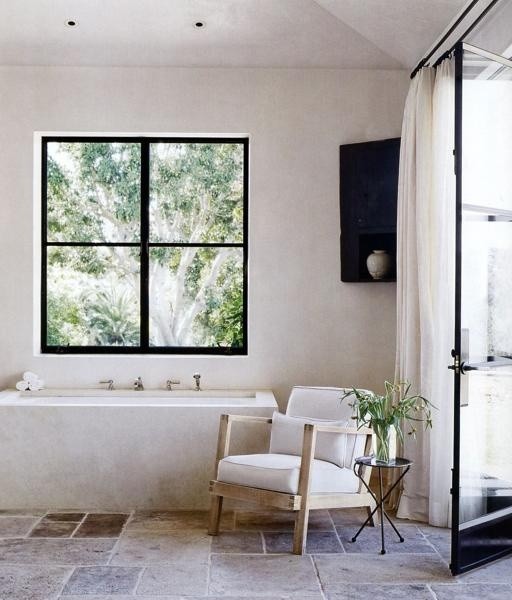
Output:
[334,382,437,464]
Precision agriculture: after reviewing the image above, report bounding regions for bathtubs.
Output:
[0,387,280,512]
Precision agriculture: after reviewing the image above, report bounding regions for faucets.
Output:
[132,378,145,390]
[192,372,203,392]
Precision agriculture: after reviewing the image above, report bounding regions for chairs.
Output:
[207,386,387,554]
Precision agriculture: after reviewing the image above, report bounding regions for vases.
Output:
[365,248,391,281]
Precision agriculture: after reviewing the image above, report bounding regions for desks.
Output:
[351,453,410,546]
